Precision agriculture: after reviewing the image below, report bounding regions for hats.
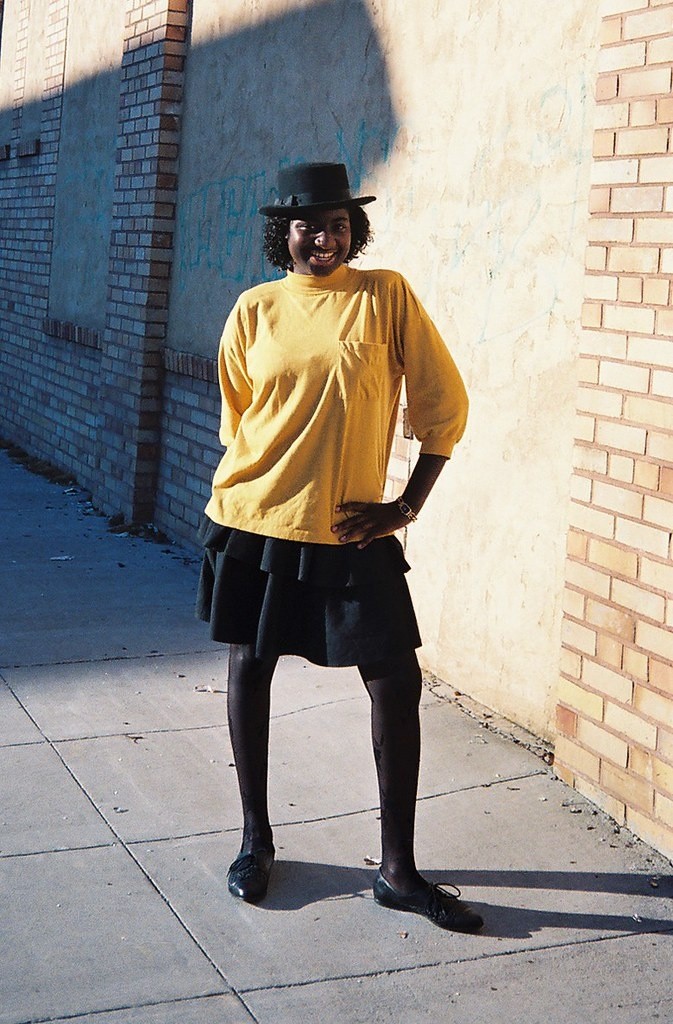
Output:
[258,164,376,219]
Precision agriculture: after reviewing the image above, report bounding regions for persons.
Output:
[194,162,485,933]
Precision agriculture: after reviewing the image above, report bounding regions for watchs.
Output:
[397,496,418,523]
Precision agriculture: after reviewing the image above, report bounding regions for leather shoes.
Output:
[372,866,484,933]
[225,843,276,902]
